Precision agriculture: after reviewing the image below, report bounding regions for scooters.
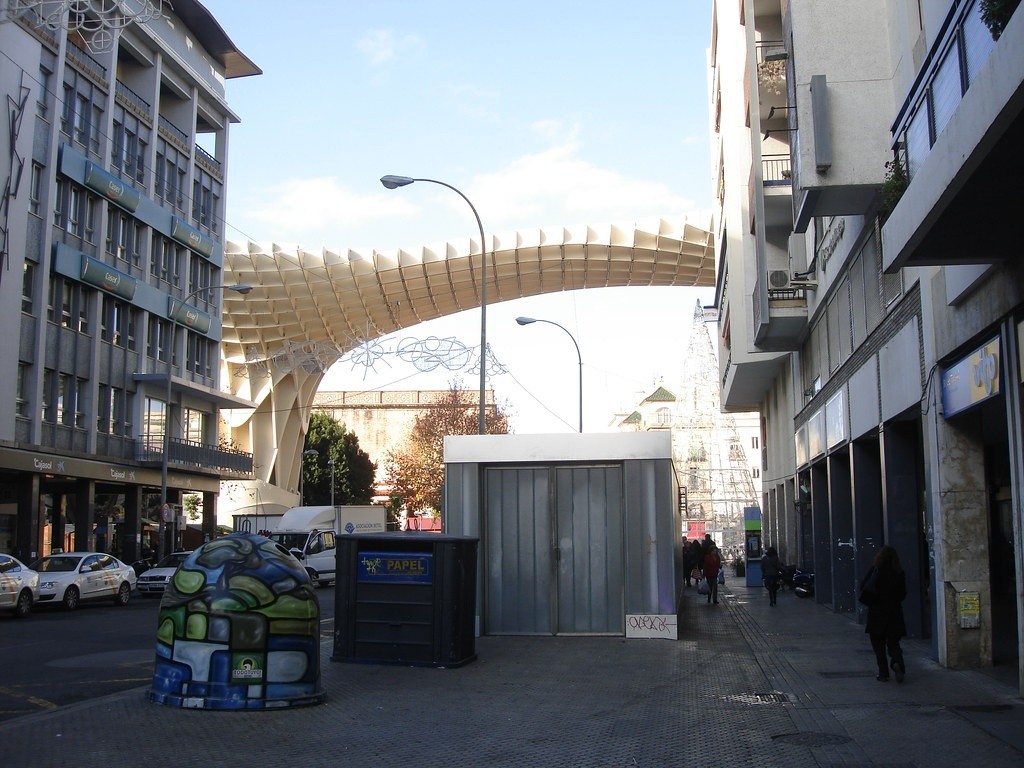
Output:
[792,568,814,598]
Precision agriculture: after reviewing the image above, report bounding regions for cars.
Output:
[0,553,41,617]
[137,550,195,592]
[28,551,137,612]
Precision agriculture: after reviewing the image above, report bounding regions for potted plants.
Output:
[881,161,907,209]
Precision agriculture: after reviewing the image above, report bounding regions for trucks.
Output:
[270,505,387,586]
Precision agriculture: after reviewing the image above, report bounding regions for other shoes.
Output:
[876,674,889,683]
[893,662,904,684]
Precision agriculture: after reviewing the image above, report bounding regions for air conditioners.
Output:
[767,270,791,290]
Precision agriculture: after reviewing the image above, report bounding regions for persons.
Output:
[307,529,324,553]
[861,545,908,681]
[760,548,782,605]
[681,534,724,603]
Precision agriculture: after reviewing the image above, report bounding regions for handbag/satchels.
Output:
[718,569,725,584]
[692,564,701,579]
[698,577,712,595]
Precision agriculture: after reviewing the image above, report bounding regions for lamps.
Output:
[763,128,798,141]
[765,50,789,62]
[768,106,796,119]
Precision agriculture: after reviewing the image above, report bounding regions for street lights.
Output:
[301,449,319,508]
[327,460,335,506]
[515,315,583,434]
[381,173,487,436]
